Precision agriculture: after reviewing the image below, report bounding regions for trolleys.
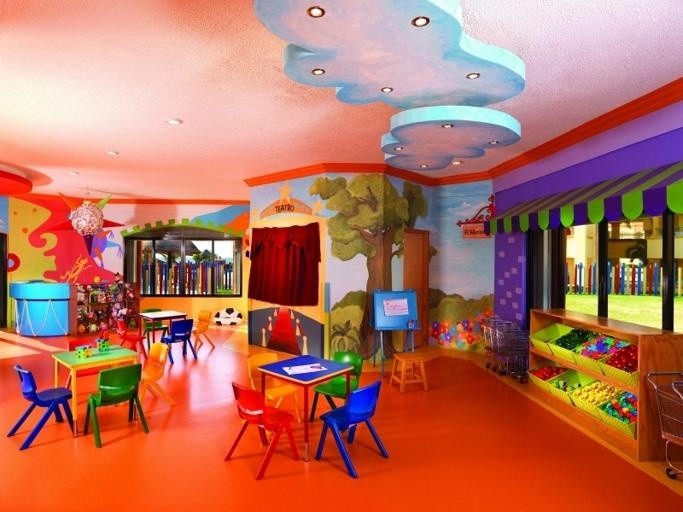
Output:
[479,316,529,385]
[644,368,682,484]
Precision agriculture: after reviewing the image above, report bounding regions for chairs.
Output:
[225,351,389,480]
[6,339,175,451]
[113,308,216,365]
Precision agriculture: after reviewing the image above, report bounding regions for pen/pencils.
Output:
[289,366,291,370]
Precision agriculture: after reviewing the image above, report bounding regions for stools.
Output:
[390,351,428,393]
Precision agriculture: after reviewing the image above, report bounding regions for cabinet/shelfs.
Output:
[529,308,682,462]
